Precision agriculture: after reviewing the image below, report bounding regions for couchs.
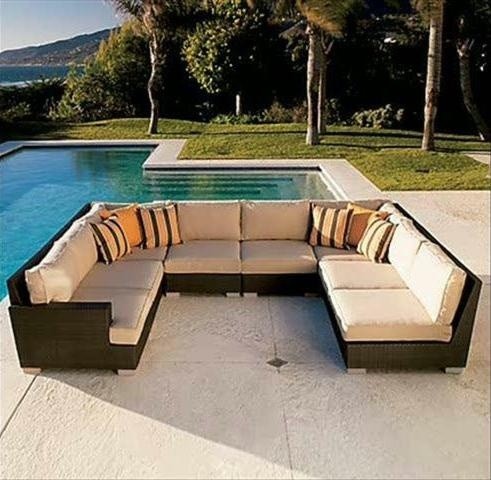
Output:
[6,198,483,376]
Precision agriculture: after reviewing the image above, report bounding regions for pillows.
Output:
[307,201,397,265]
[88,202,184,266]
